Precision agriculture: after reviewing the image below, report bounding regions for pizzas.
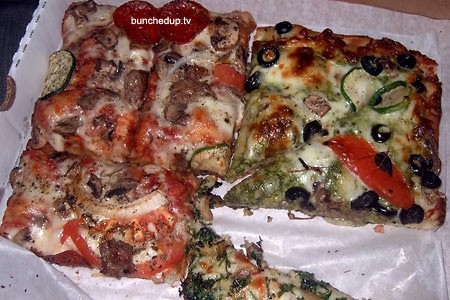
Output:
[179,223,358,300]
[225,22,447,230]
[0,0,256,283]
